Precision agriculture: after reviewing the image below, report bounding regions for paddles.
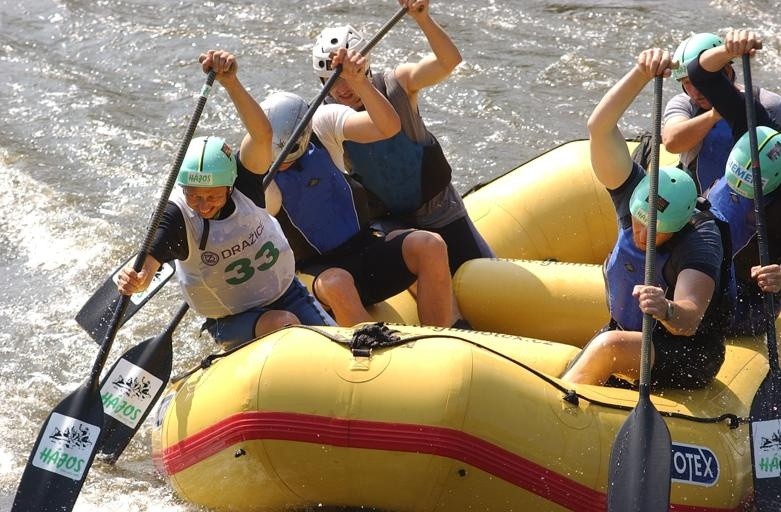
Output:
[739,54,781,512]
[607,61,678,510]
[102,0,424,469]
[75,250,174,344]
[9,66,218,512]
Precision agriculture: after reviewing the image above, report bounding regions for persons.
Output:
[237,46,456,331]
[687,27,781,338]
[308,2,498,280]
[558,44,730,395]
[659,31,780,202]
[115,49,337,354]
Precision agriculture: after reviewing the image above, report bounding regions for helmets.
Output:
[723,125,781,201]
[176,135,241,188]
[311,24,372,79]
[257,90,314,165]
[626,166,698,234]
[672,31,731,84]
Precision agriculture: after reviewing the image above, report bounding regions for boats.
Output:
[150,137,781,512]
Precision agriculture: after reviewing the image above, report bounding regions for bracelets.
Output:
[665,297,674,322]
